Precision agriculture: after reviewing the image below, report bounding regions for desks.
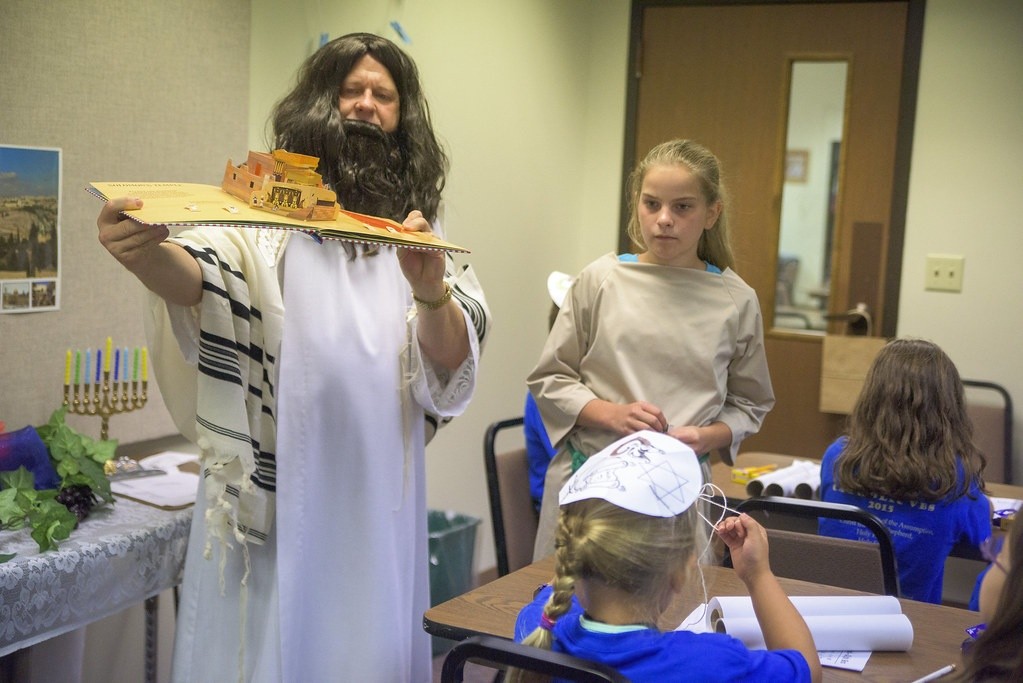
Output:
[424,556,993,683]
[1,431,201,683]
[702,450,1023,566]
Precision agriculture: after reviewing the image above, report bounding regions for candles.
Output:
[133,347,139,381]
[123,348,130,382]
[104,336,112,371]
[85,350,91,385]
[142,347,148,383]
[96,349,101,384]
[63,349,71,385]
[114,348,120,382]
[76,349,81,385]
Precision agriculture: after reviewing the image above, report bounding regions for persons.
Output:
[99,31,489,683]
[819,339,993,608]
[967,528,1008,611]
[525,270,579,522]
[528,137,775,561]
[511,431,821,683]
[922,498,1023,683]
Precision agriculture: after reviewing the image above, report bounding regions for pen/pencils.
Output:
[912,664,956,683]
[742,464,778,476]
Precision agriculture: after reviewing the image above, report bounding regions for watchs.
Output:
[413,281,453,311]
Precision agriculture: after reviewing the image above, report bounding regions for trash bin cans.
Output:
[428,510,482,658]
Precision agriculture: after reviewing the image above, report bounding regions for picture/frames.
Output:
[1,146,63,313]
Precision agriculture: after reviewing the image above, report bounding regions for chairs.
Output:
[723,496,898,595]
[483,417,549,577]
[958,381,1013,484]
[440,637,624,683]
